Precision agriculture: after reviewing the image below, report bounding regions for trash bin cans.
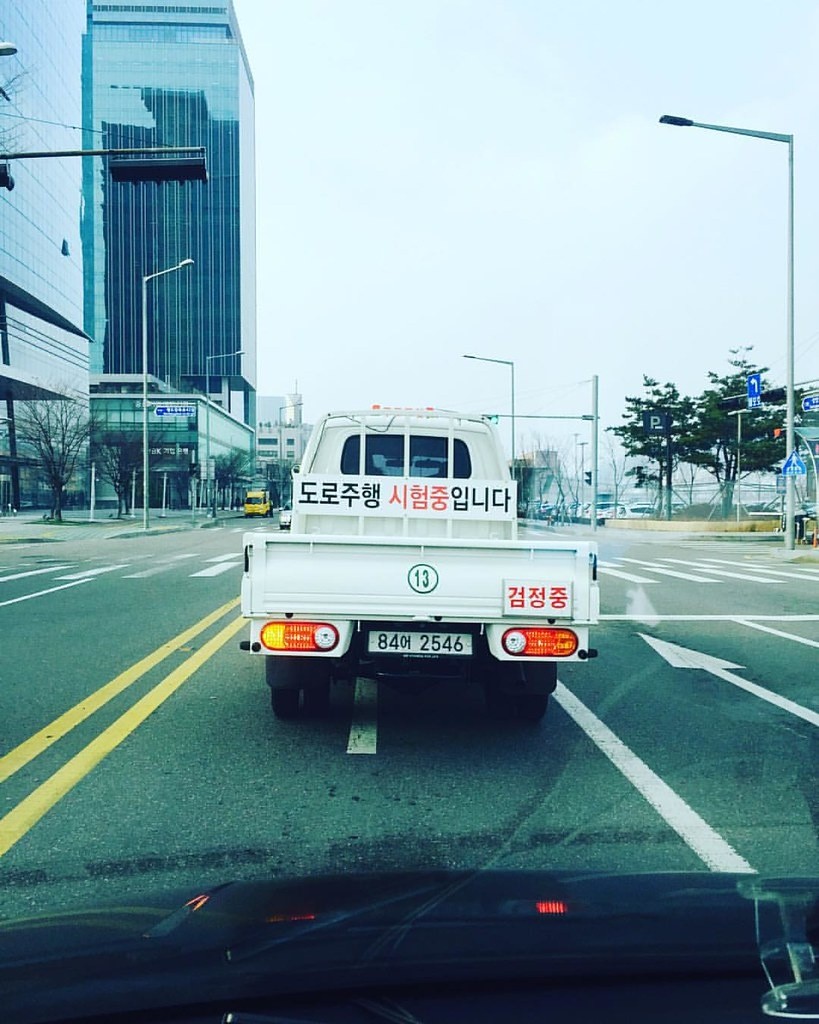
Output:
[793,509,808,544]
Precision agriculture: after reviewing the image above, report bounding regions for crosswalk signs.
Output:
[783,449,807,476]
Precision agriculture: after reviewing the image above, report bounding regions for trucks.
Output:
[244,492,273,517]
[238,406,601,719]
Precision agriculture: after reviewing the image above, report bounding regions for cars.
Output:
[528,503,581,523]
[277,501,292,531]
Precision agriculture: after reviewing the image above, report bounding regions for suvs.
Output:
[578,502,619,524]
[620,504,671,519]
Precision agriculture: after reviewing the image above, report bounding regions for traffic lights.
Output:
[717,400,740,410]
[491,416,498,424]
[584,471,592,486]
[760,389,784,402]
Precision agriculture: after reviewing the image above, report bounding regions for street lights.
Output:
[659,113,795,553]
[279,404,305,508]
[142,259,196,526]
[206,351,247,517]
[463,354,515,480]
